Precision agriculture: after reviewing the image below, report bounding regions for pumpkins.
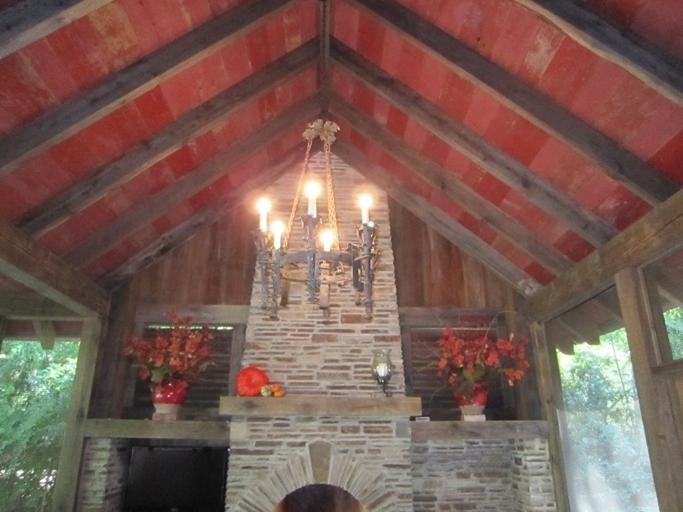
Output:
[236,367,285,398]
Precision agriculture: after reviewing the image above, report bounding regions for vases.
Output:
[371,344,391,384]
[146,379,188,420]
[456,381,488,422]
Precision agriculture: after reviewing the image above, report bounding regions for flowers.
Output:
[113,309,220,388]
[411,308,533,406]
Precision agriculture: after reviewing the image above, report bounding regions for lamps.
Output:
[246,69,381,320]
